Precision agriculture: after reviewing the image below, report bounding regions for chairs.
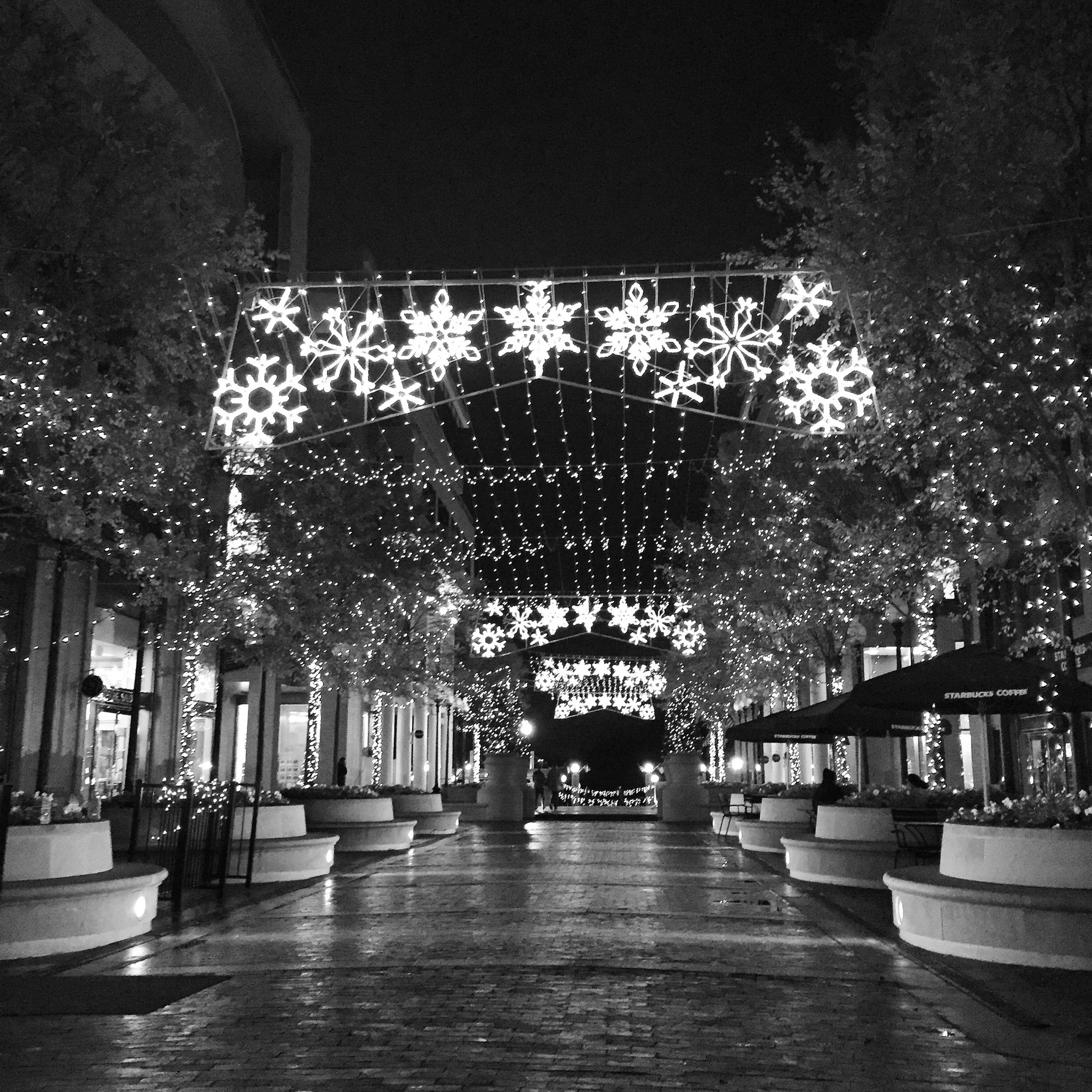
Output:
[806,811,817,834]
[717,793,754,840]
[743,794,768,814]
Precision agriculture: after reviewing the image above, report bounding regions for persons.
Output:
[335,757,349,787]
[811,768,846,816]
[533,764,547,810]
[547,764,561,811]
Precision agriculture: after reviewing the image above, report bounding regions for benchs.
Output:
[890,808,958,869]
